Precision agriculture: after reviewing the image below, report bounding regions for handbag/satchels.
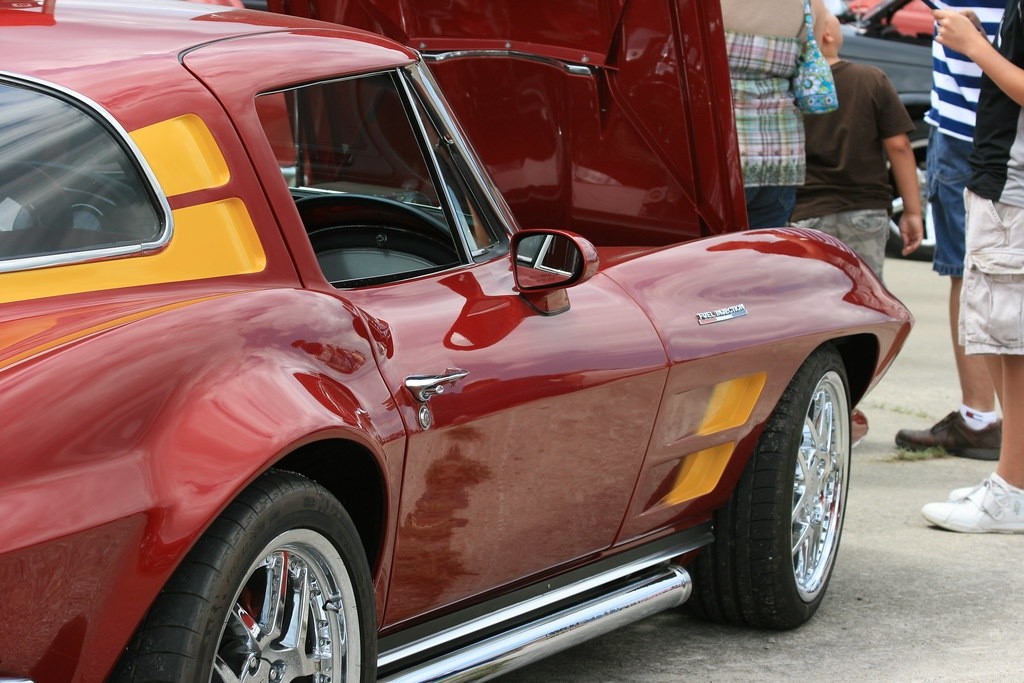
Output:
[789,0,842,114]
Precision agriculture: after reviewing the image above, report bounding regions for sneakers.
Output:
[895,410,1002,460]
[920,476,1023,536]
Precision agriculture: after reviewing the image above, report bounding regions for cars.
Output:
[832,0,939,108]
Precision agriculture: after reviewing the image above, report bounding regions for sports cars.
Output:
[0,0,916,683]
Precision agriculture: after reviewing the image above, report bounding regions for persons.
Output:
[790,0,1024,534]
[720,0,826,230]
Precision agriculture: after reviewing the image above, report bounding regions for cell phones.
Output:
[923,0,940,11]
